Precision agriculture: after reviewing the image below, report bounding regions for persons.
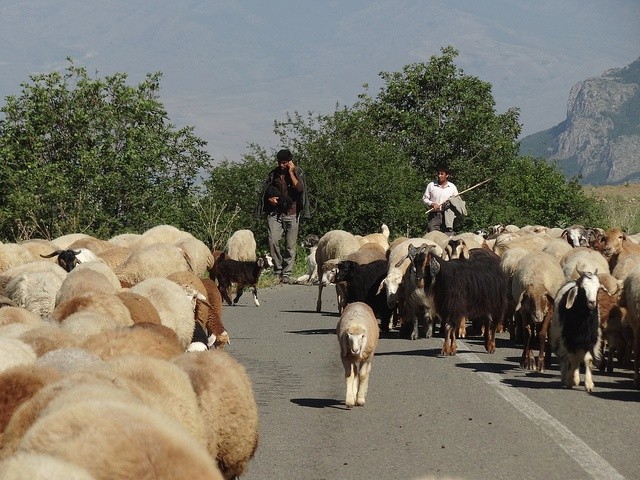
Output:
[421,165,459,233]
[257,149,311,284]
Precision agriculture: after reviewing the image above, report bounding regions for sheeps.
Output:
[512,250,566,374]
[0,224,275,480]
[315,230,361,313]
[560,246,625,375]
[322,249,391,338]
[497,248,530,347]
[549,265,613,394]
[336,301,380,409]
[600,226,640,273]
[376,237,444,340]
[355,223,605,261]
[612,254,640,385]
[414,243,509,357]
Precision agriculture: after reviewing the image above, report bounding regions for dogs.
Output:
[298,233,320,286]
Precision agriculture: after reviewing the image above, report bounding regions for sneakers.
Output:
[281,276,290,283]
[273,273,282,279]
